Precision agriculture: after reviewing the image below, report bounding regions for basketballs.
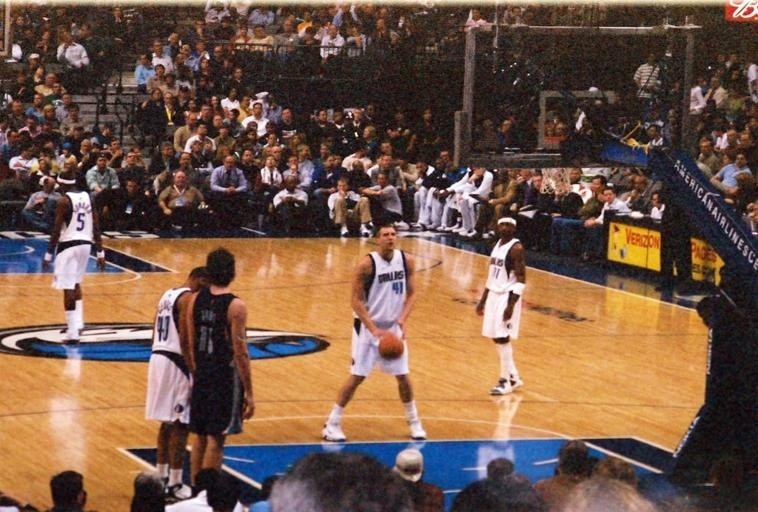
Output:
[379,336,404,360]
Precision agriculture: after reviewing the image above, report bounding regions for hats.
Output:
[392,446,425,483]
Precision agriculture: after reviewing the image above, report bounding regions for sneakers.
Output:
[410,221,496,242]
[340,225,351,237]
[319,420,347,442]
[60,333,81,343]
[162,483,193,503]
[157,474,169,486]
[510,370,523,390]
[358,224,372,237]
[60,323,86,333]
[653,280,695,293]
[489,376,512,395]
[405,417,427,439]
